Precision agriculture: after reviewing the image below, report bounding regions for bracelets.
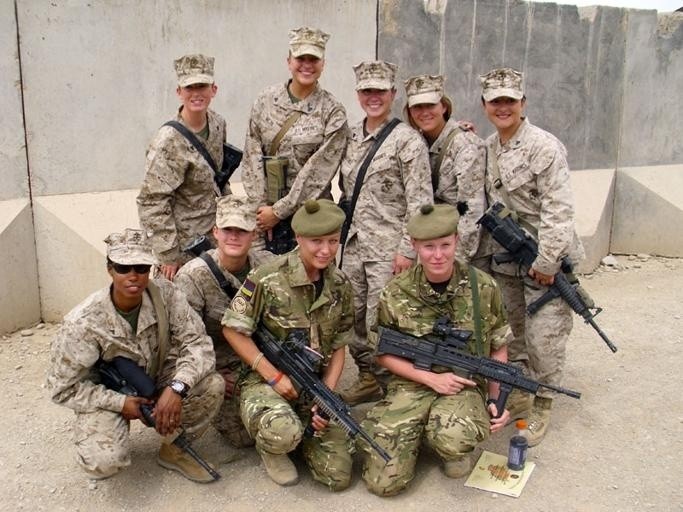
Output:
[251,352,285,386]
[486,399,498,404]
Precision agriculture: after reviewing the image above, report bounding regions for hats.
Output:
[288,26,330,59]
[402,75,444,107]
[173,55,214,87]
[406,205,460,240]
[352,60,397,91]
[103,228,153,266]
[291,199,346,237]
[216,194,257,231]
[479,68,524,102]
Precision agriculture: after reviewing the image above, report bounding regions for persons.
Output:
[402,74,488,275]
[220,197,355,491]
[242,27,347,252]
[137,53,234,279]
[362,204,514,497]
[461,68,581,443]
[48,228,224,485]
[174,194,280,448]
[334,61,431,405]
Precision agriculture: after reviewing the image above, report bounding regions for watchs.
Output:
[169,380,188,398]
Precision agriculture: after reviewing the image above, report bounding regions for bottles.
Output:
[507,417,527,471]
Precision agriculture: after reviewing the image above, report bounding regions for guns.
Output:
[477,201,595,330]
[216,142,244,190]
[263,155,299,256]
[92,356,222,482]
[378,318,581,424]
[250,324,392,463]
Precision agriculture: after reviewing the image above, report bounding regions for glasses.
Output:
[109,263,151,273]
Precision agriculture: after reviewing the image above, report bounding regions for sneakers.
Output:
[156,443,215,482]
[505,391,552,448]
[339,380,384,406]
[444,457,470,478]
[255,446,299,487]
